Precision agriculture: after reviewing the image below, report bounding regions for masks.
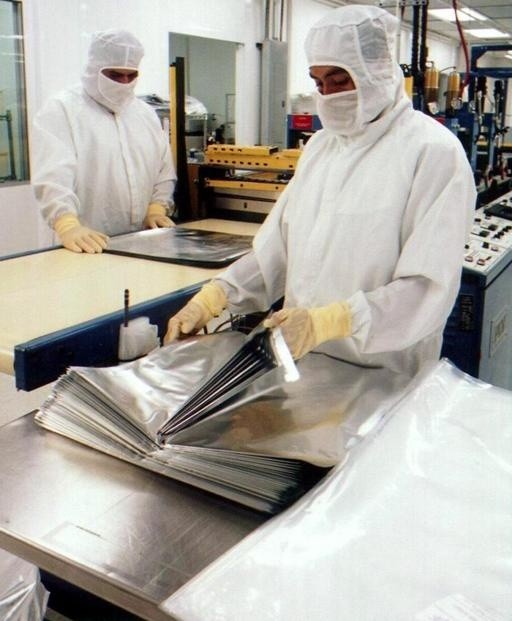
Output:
[316,90,358,133]
[101,72,138,104]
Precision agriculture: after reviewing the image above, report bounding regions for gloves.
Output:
[162,280,229,348]
[144,202,176,232]
[262,300,356,363]
[52,211,110,256]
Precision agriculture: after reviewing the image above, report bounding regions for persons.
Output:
[162,2,479,377]
[28,28,178,255]
